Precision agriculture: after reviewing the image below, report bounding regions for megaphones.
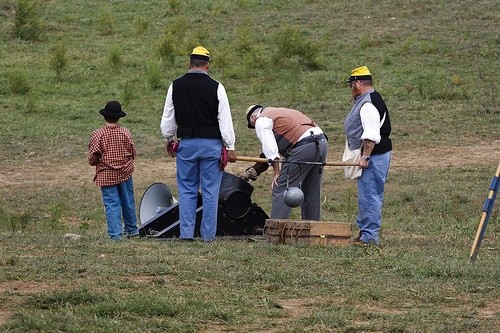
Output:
[139,182,178,224]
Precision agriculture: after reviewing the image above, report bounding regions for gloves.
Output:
[239,169,258,182]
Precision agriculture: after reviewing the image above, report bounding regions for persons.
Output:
[87,101,139,240]
[245,105,328,221]
[160,46,237,239]
[344,66,392,245]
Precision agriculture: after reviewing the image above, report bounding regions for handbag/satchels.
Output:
[341,145,363,180]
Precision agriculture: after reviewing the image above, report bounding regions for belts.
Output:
[291,133,325,149]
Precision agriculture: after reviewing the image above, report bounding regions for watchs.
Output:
[362,156,369,161]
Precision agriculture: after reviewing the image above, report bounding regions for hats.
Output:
[190,46,210,62]
[245,104,262,129]
[99,101,126,117]
[351,65,372,80]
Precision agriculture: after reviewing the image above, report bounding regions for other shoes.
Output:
[352,233,366,245]
